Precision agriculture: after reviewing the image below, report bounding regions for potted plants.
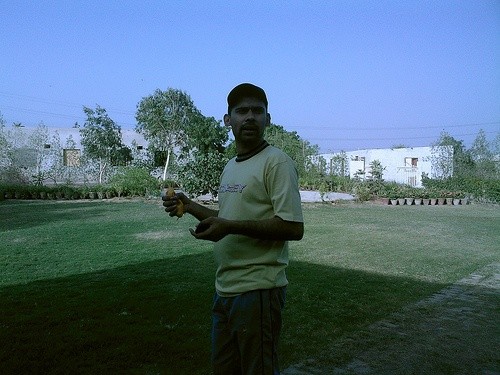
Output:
[389,187,468,206]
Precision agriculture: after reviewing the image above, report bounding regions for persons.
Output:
[162,83,306,375]
